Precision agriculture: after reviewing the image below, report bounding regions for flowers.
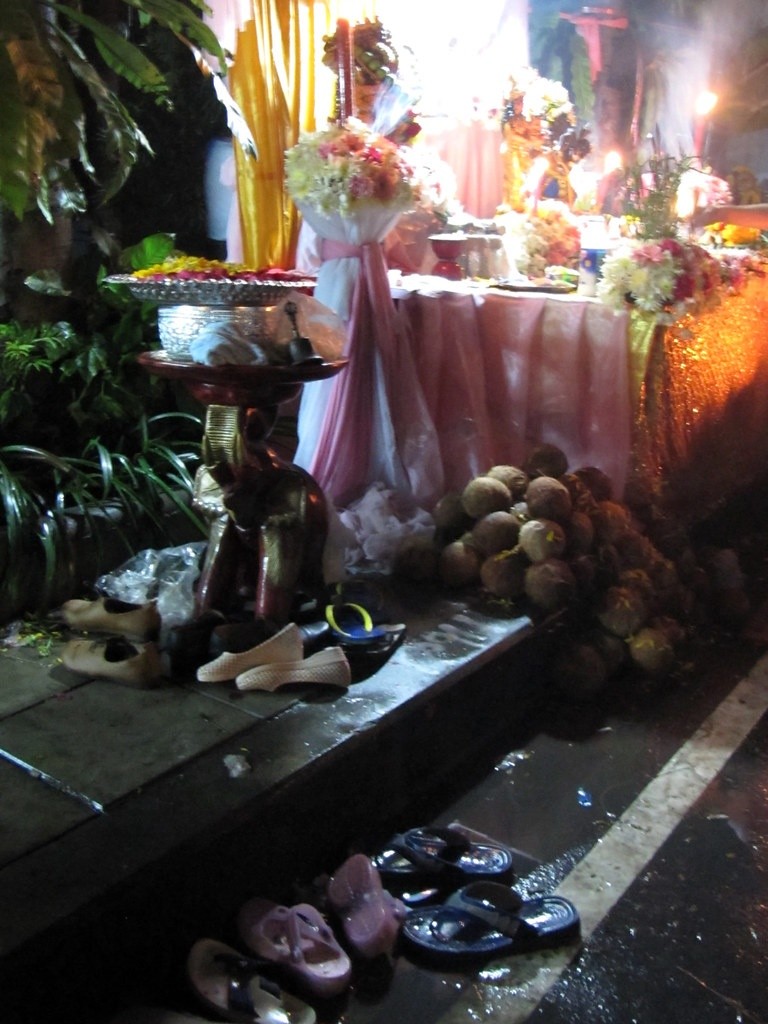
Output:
[589,238,757,317]
[506,66,574,133]
[281,122,433,215]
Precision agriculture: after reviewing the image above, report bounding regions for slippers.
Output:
[401,881,581,955]
[370,826,514,884]
[234,896,353,995]
[185,937,319,1022]
[326,853,405,956]
[327,601,386,647]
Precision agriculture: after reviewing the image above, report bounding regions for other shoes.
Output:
[234,647,351,691]
[60,634,148,687]
[60,596,162,644]
[198,622,303,683]
[206,619,330,661]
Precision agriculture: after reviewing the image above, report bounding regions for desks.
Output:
[139,351,352,622]
[384,267,768,529]
